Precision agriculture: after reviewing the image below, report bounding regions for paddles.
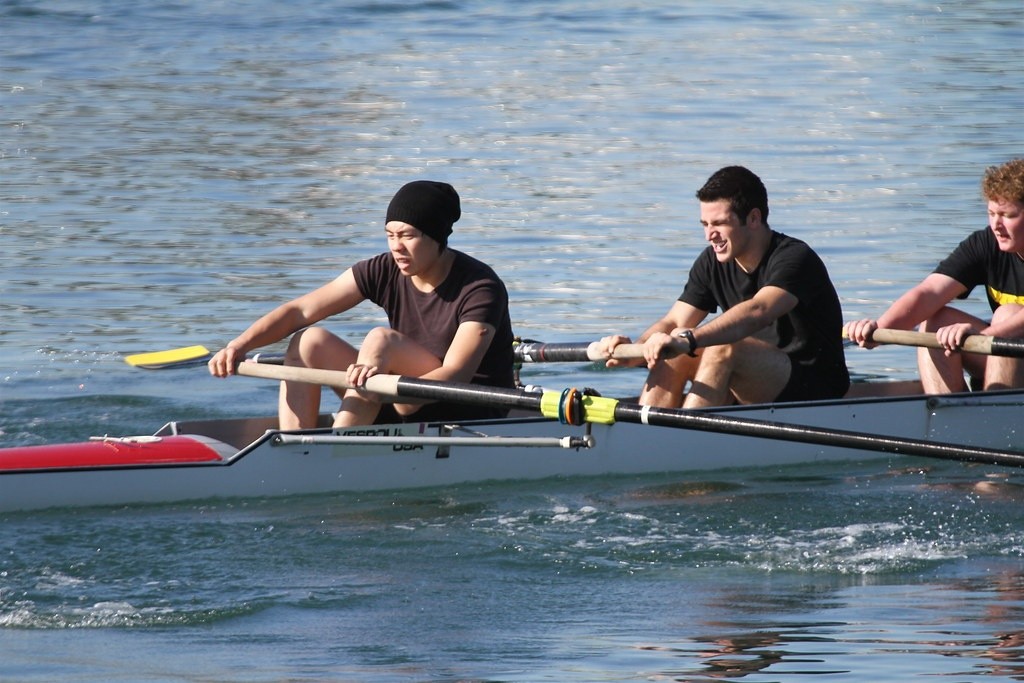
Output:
[843,322,1023,358]
[123,336,673,371]
[233,359,1023,472]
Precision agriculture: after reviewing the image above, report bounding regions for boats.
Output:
[0,376,1024,514]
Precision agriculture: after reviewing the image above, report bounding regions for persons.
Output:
[208,181,517,429]
[595,165,850,409]
[845,159,1024,396]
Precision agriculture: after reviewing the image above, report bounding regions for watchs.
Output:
[678,330,698,358]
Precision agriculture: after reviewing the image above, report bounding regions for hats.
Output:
[385,180,461,245]
